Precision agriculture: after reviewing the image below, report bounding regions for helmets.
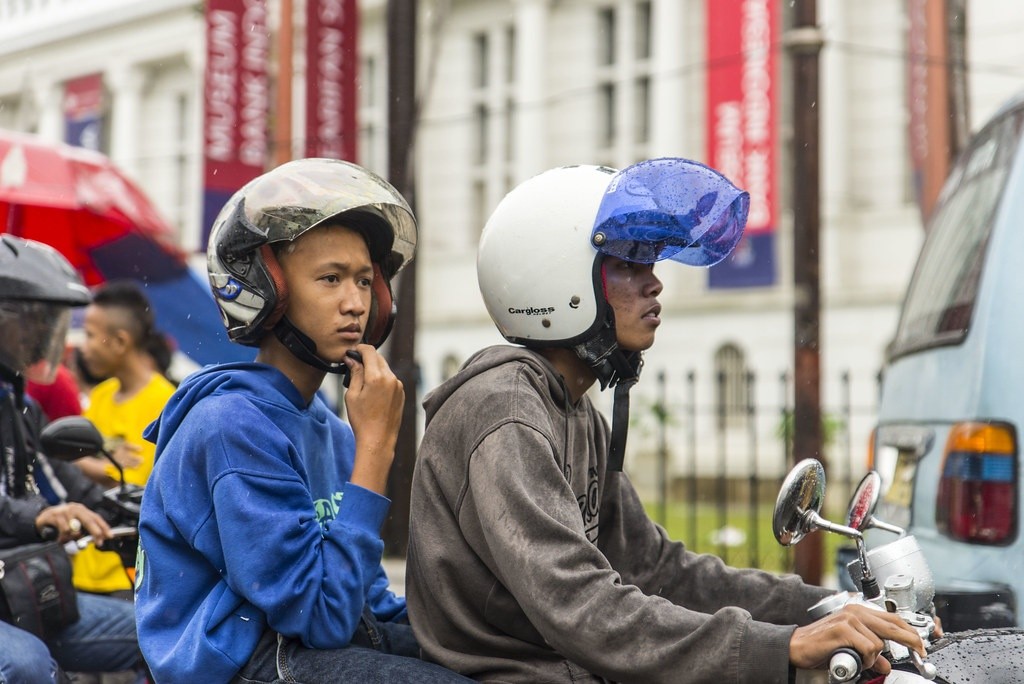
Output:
[476,165,699,349]
[0,232,92,313]
[206,159,404,350]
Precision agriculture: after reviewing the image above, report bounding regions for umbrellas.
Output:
[0,129,187,289]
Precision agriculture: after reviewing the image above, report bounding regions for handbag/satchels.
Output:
[0,541,81,642]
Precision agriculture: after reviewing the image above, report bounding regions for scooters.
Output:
[772,458,1024,684]
[41,418,147,571]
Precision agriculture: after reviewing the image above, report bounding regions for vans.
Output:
[836,86,1023,634]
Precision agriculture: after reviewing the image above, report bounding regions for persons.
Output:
[405,157,943,684]
[0,233,146,684]
[68,280,179,684]
[135,157,477,684]
